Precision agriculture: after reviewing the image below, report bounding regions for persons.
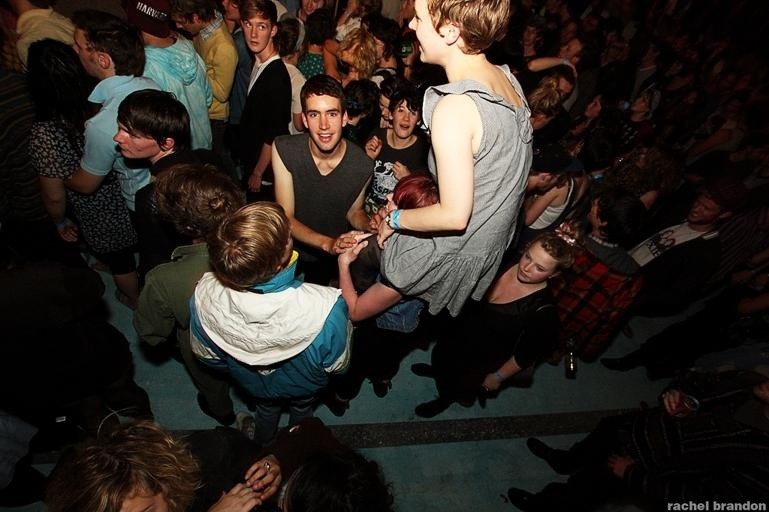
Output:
[285,1,450,137]
[412,233,575,417]
[1,253,396,510]
[191,201,354,444]
[376,0,534,322]
[361,78,400,145]
[342,78,380,151]
[488,1,765,512]
[0,0,291,286]
[271,74,379,287]
[133,164,257,425]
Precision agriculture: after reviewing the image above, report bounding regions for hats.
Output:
[133,1,171,37]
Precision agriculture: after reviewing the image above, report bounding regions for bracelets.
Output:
[392,209,400,230]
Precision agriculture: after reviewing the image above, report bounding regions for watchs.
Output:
[385,210,394,230]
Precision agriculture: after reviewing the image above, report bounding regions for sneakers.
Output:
[197,357,632,512]
